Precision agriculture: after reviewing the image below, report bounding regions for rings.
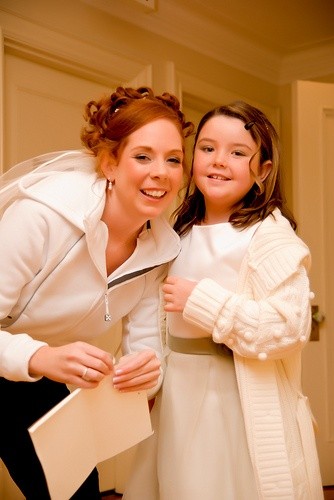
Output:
[82,367,88,378]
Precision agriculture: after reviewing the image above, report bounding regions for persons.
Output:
[122,102,324,500]
[0,86,197,500]
